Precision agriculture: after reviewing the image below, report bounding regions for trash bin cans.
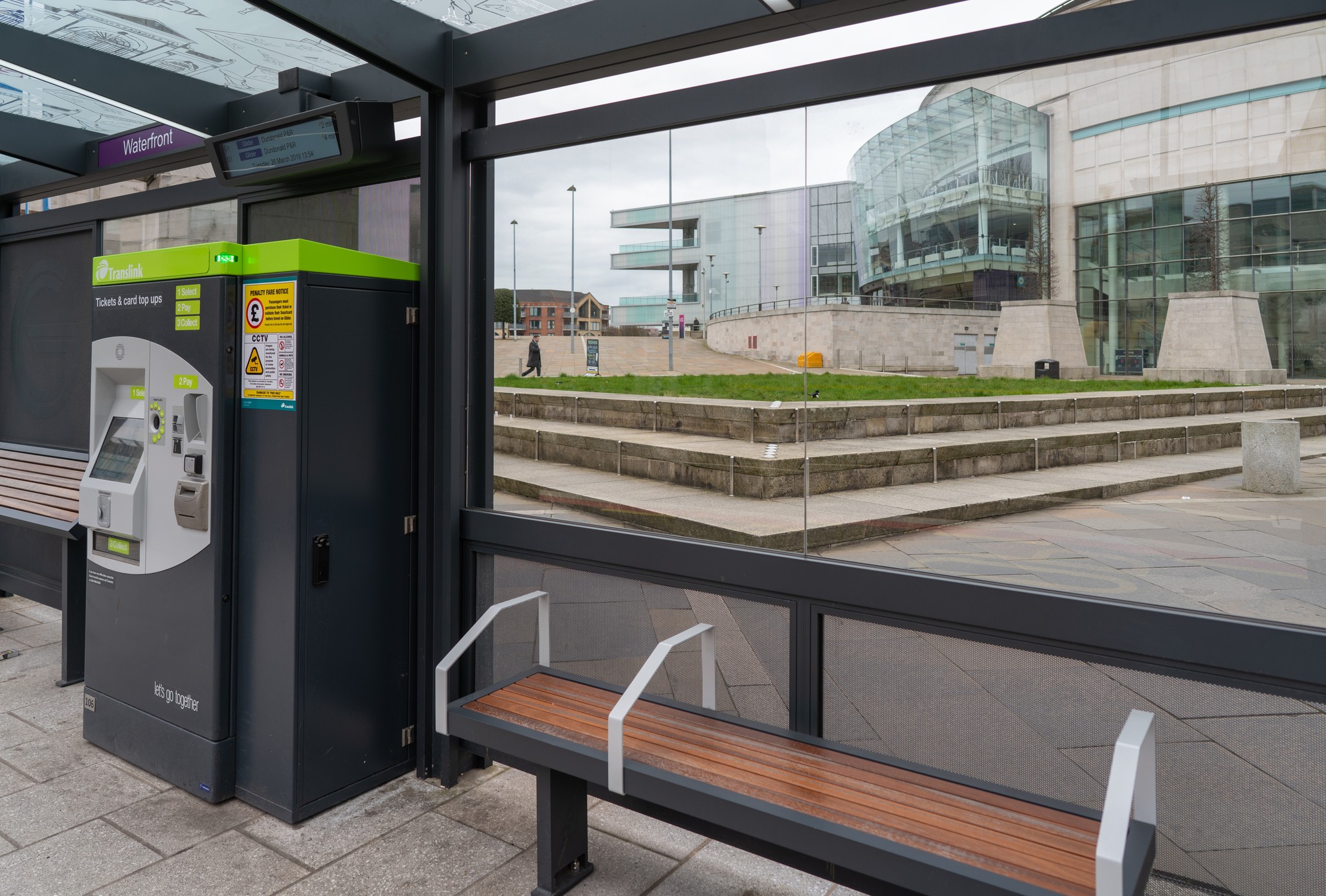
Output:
[1035,359,1060,380]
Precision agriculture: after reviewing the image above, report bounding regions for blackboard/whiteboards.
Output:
[662,320,669,339]
[586,339,599,373]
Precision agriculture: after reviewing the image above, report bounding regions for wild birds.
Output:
[806,389,821,400]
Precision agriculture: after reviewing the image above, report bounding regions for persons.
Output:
[693,318,699,331]
[522,335,541,376]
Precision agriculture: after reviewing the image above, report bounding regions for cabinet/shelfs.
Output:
[1115,348,1149,375]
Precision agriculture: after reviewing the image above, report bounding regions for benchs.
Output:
[430,588,1158,896]
[864,245,1040,279]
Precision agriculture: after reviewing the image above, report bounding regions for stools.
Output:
[1015,244,1021,257]
[951,246,957,259]
[991,242,996,251]
[930,251,936,263]
[941,248,946,260]
[965,246,971,256]
[975,243,979,255]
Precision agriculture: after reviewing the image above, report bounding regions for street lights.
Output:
[773,285,780,309]
[706,254,716,315]
[753,225,767,304]
[510,219,518,343]
[722,272,730,316]
[566,185,577,353]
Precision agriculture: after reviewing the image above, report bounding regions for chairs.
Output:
[1304,359,1317,376]
